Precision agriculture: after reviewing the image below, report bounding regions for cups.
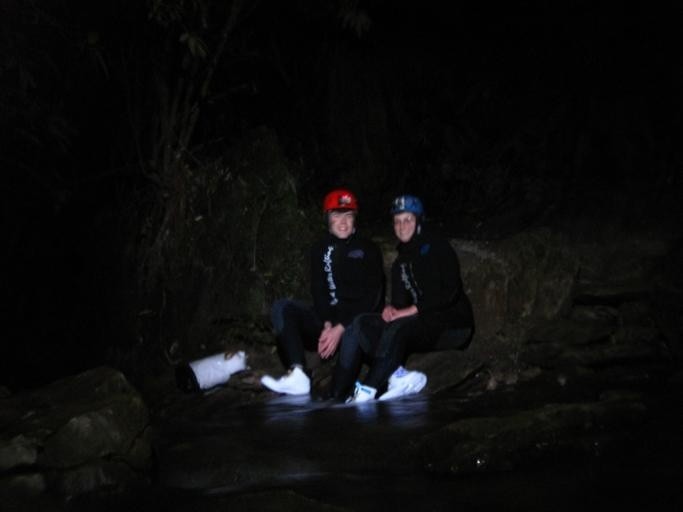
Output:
[389,216,414,223]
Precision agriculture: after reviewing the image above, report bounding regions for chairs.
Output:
[343,365,428,404]
[260,367,312,397]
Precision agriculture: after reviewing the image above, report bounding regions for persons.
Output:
[344,195,475,404]
[261,189,388,396]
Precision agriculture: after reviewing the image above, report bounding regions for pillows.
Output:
[322,188,358,212]
[389,194,424,217]
